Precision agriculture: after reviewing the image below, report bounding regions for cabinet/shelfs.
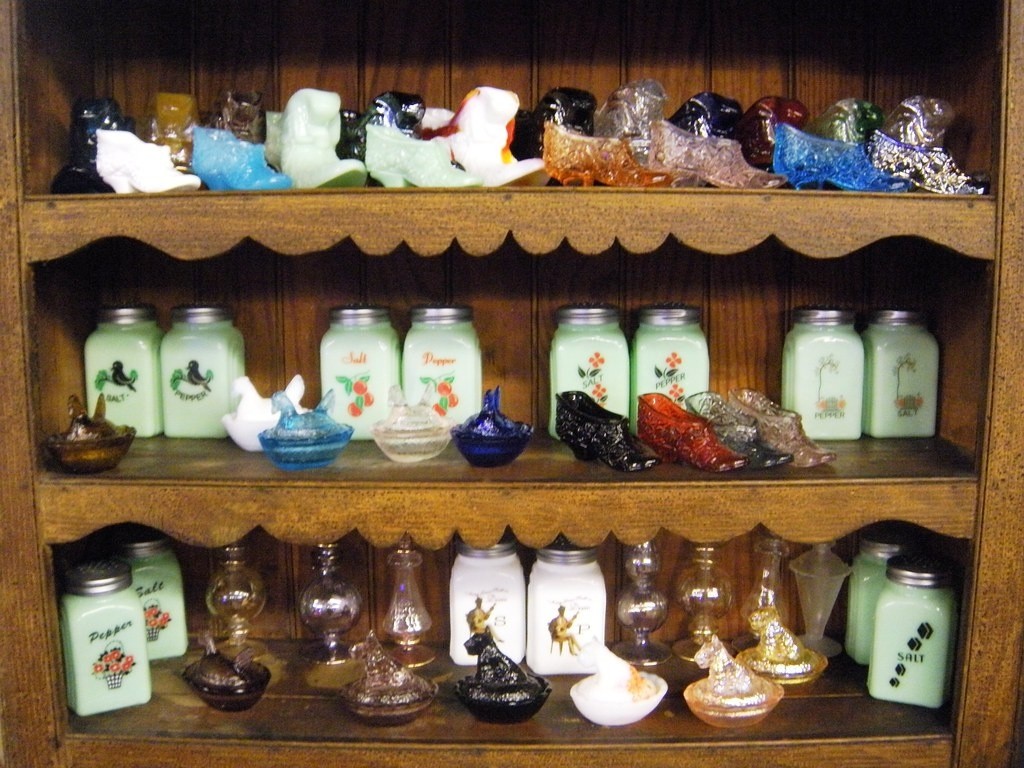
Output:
[3,0,1024,766]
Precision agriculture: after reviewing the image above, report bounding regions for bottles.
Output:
[613,536,673,663]
[83,304,165,437]
[117,533,190,660]
[159,305,247,439]
[296,540,361,664]
[842,533,918,663]
[861,308,937,437]
[674,541,736,662]
[400,303,481,425]
[319,305,402,441]
[382,533,438,664]
[57,560,152,716]
[787,538,851,655]
[206,535,269,660]
[548,302,630,440]
[449,535,526,665]
[628,304,710,436]
[730,532,789,651]
[781,305,862,440]
[866,557,960,708]
[526,536,606,675]
